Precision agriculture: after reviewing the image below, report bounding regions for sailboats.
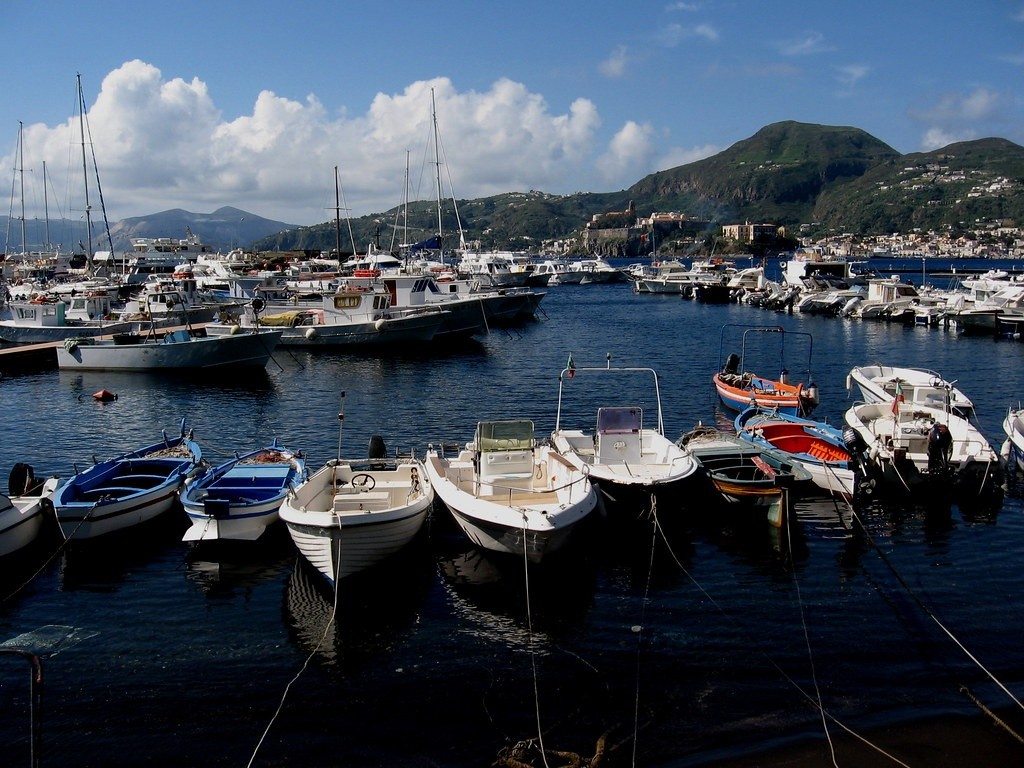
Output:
[1,81,1024,373]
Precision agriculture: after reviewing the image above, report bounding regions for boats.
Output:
[713,323,813,417]
[424,419,598,555]
[0,462,67,557]
[278,389,435,583]
[179,437,314,541]
[52,416,212,541]
[549,367,1024,527]
